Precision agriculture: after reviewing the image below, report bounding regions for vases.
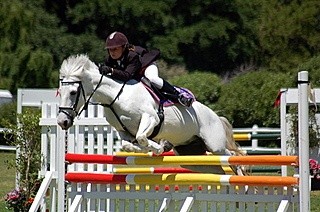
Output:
[311,177,320,191]
[14,206,20,212]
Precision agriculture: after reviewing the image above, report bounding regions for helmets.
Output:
[104,32,127,49]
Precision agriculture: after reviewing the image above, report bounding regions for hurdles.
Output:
[28,71,311,212]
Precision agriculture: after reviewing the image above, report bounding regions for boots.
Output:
[162,78,194,107]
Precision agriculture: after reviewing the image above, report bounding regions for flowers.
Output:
[309,158,320,177]
[5,190,34,210]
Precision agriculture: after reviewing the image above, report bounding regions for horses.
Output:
[57,53,258,209]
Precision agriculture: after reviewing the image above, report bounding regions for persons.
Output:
[99,31,195,108]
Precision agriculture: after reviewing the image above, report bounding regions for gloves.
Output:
[99,63,112,75]
